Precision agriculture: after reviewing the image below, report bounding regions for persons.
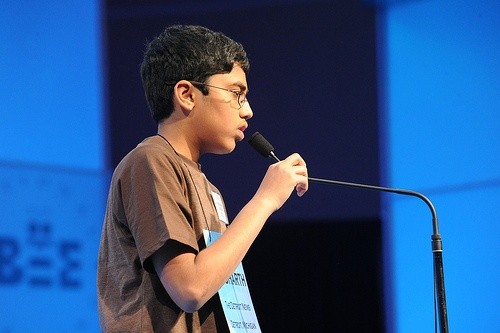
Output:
[96,24,309,332]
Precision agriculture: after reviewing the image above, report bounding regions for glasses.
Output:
[190,81,246,107]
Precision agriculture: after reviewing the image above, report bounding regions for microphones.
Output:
[249,131,448,333]
